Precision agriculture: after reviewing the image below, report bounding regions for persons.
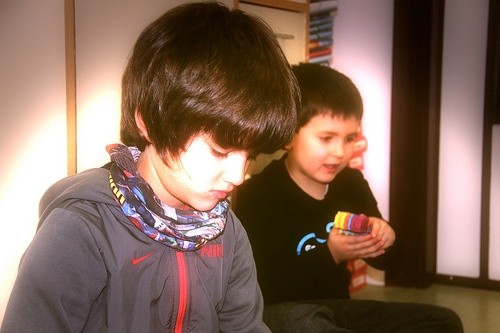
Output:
[1,0,303,333]
[234,62,419,301]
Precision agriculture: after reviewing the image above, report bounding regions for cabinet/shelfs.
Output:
[234,1,333,73]
